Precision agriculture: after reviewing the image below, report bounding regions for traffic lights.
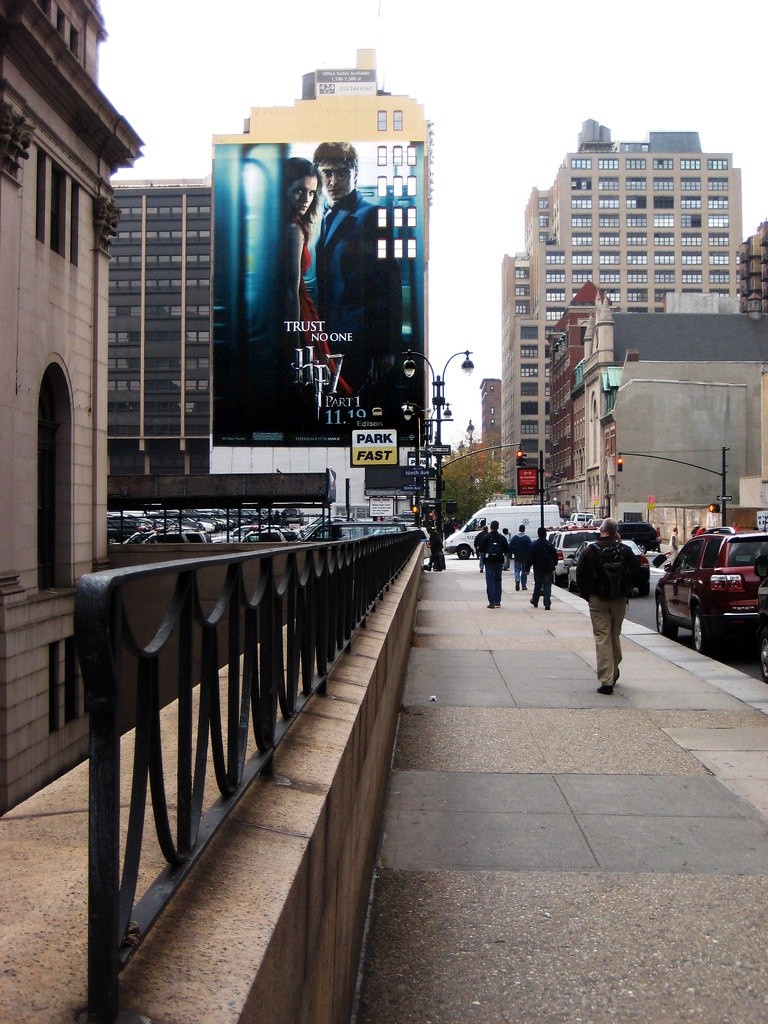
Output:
[412,505,419,514]
[709,504,715,512]
[523,453,528,467]
[516,451,522,465]
[617,458,623,471]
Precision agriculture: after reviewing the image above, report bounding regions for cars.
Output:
[107,507,435,559]
[567,539,651,599]
[546,512,605,549]
[753,554,768,685]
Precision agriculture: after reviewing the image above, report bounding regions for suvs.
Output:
[654,526,768,654]
[615,520,662,556]
[551,529,621,587]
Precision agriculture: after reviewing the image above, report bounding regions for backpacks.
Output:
[590,544,628,600]
[484,534,501,568]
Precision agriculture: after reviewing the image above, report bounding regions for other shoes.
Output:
[597,685,614,695]
[523,588,527,590]
[530,598,538,607]
[496,601,501,606]
[515,581,520,591]
[613,669,620,686]
[486,604,495,608]
[544,605,550,610]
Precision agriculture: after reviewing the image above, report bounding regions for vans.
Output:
[443,500,562,561]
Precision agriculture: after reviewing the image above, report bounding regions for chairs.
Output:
[731,544,756,567]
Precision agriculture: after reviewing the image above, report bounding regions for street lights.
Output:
[467,419,475,445]
[402,400,453,533]
[400,349,475,570]
[407,423,433,525]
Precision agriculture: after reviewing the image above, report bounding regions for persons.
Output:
[564,523,577,531]
[525,527,558,610]
[576,518,640,693]
[443,519,454,540]
[691,524,706,538]
[502,528,511,571]
[279,157,352,396]
[474,526,489,573]
[314,142,402,405]
[508,525,531,591]
[733,522,740,533]
[653,527,661,552]
[669,527,679,565]
[423,527,442,571]
[479,521,509,609]
